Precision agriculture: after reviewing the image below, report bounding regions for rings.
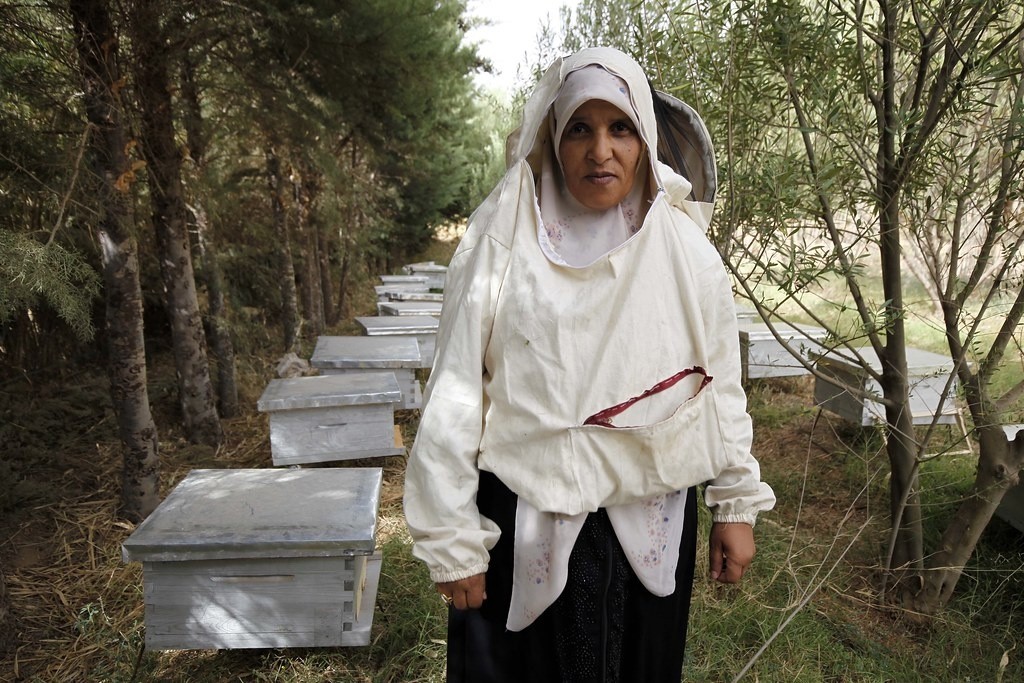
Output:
[439,592,453,606]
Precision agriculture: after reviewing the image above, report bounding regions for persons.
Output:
[399,43,777,683]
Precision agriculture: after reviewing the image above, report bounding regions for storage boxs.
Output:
[257,372,406,468]
[381,303,442,316]
[409,265,448,288]
[739,322,829,378]
[310,335,423,410]
[123,466,386,652]
[378,275,429,285]
[735,303,758,323]
[995,425,1024,529]
[808,345,974,426]
[353,316,439,370]
[374,285,429,301]
[402,261,435,274]
[384,291,443,303]
